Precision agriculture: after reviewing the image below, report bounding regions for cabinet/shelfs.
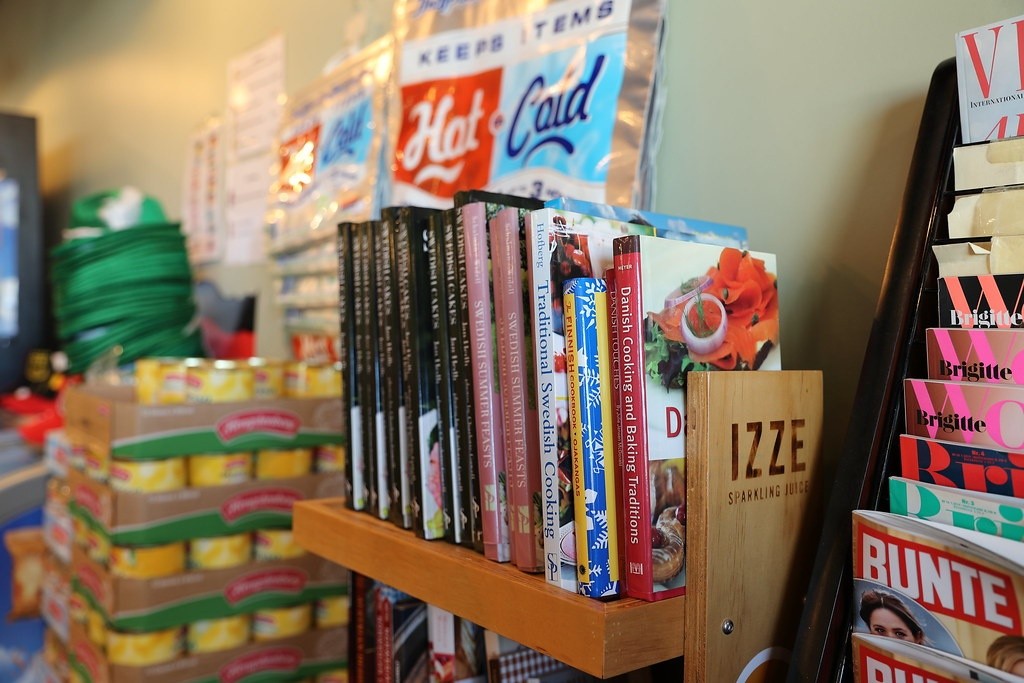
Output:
[292,370,824,683]
[31,387,353,682]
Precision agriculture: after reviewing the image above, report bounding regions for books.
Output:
[851,15,1024,683]
[336,189,782,683]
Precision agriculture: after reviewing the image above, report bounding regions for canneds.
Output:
[67,354,351,666]
